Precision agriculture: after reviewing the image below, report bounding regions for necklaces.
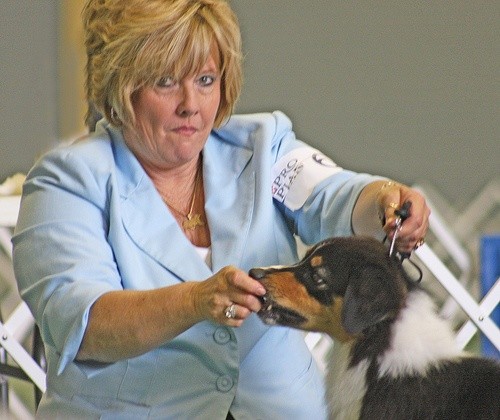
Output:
[157,166,202,230]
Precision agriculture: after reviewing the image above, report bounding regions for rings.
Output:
[224,302,235,318]
[415,238,424,250]
[384,203,398,212]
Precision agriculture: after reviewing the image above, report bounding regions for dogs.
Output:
[249,235,500,420]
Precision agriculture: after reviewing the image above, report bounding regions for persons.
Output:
[11,0,430,420]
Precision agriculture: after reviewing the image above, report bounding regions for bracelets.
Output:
[375,181,398,223]
